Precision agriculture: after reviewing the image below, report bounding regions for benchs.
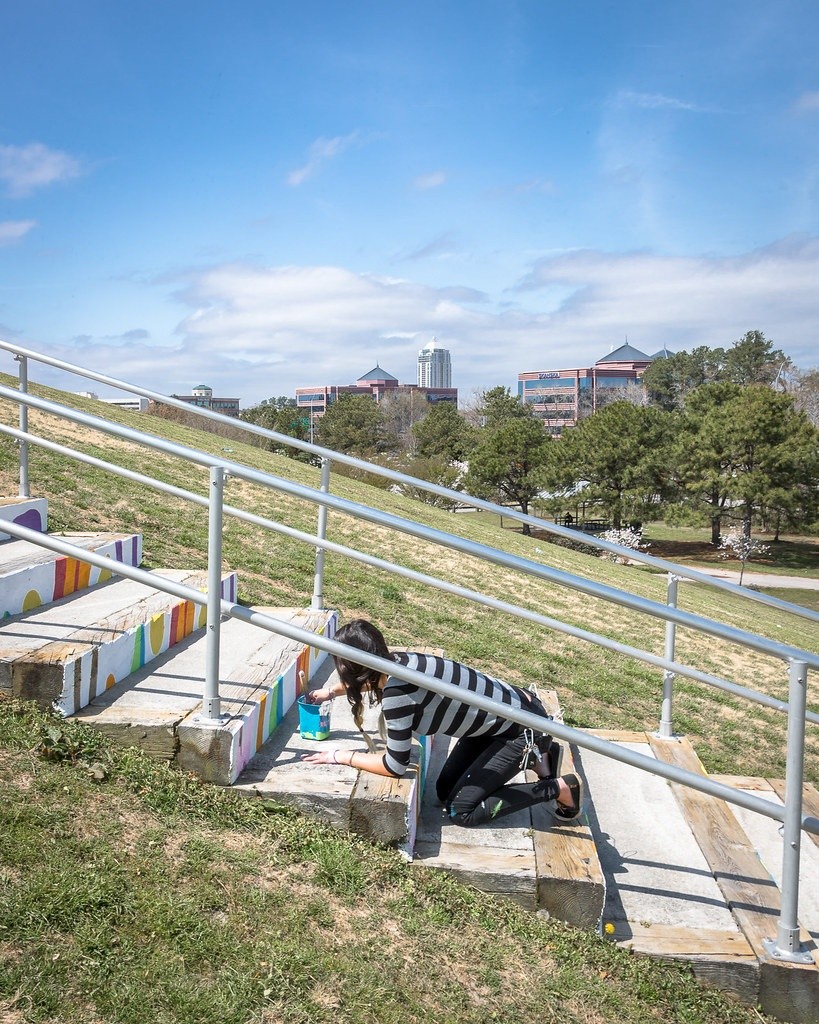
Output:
[557,521,613,530]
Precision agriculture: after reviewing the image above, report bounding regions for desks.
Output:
[584,519,608,528]
[556,516,580,525]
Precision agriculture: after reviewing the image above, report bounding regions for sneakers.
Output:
[538,742,564,780]
[554,772,584,822]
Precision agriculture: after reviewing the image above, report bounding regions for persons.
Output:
[308,619,584,827]
[565,512,573,528]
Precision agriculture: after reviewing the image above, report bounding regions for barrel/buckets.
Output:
[297,695,332,741]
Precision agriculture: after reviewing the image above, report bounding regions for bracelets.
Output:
[334,750,340,764]
[350,751,357,766]
[331,689,336,700]
[328,688,333,701]
